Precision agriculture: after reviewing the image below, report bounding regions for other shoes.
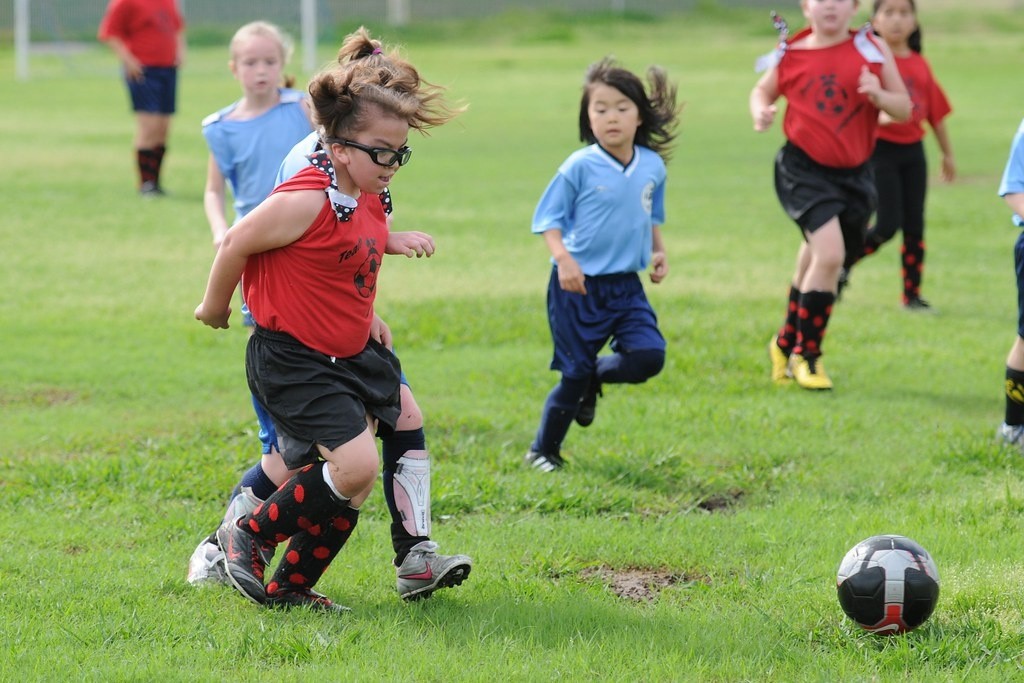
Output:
[906,297,934,313]
[140,183,165,198]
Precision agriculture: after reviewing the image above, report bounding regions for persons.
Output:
[201,21,314,335]
[997,120,1024,443]
[749,0,913,389]
[98,0,186,195]
[841,1,956,307]
[186,27,471,601]
[195,60,461,615]
[525,56,684,470]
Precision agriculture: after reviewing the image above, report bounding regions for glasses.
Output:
[323,138,412,166]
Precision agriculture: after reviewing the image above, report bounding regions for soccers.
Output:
[836,534,942,634]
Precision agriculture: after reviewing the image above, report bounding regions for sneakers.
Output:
[187,533,232,587]
[574,371,602,428]
[996,422,1024,455]
[525,447,569,474]
[263,582,355,617]
[768,333,796,384]
[787,353,834,389]
[396,540,473,604]
[217,513,278,605]
